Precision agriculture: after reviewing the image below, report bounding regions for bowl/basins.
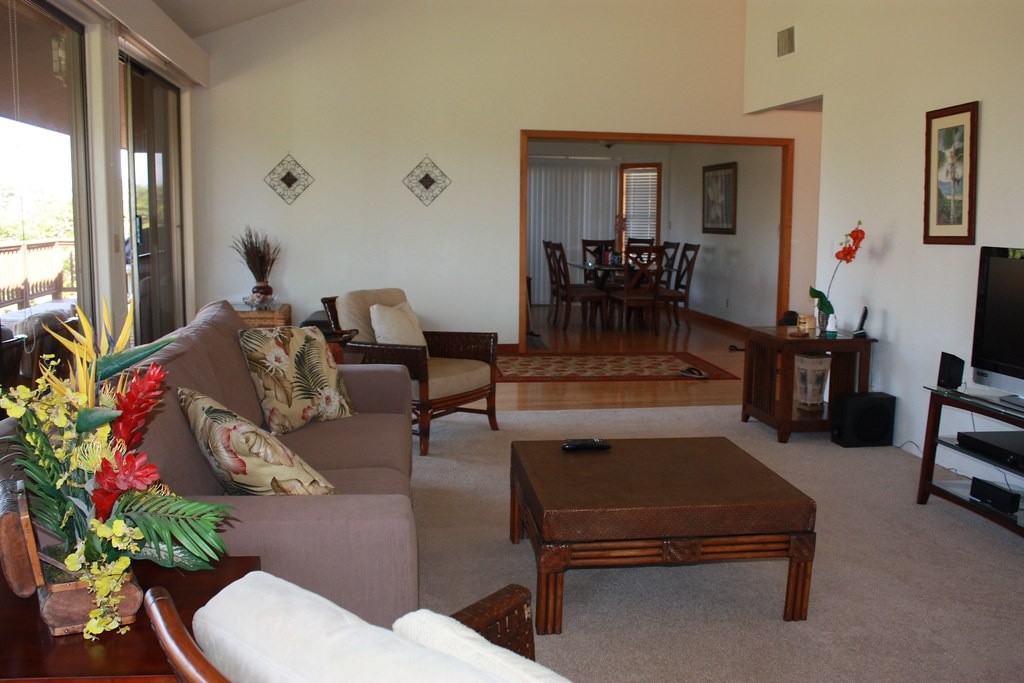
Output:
[243,295,276,307]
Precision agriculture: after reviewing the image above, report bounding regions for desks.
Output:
[742,324,878,443]
[299,311,331,330]
[571,263,679,329]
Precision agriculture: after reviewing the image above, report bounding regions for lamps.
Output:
[50,33,67,76]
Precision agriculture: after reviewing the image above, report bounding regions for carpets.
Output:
[495,352,741,382]
[409,405,1024,683]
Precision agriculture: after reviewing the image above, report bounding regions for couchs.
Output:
[0,301,418,633]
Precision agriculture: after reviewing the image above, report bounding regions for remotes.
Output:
[562,438,611,451]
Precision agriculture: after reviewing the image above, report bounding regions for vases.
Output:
[253,281,272,296]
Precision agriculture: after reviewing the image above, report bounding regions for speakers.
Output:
[936,352,964,389]
[830,392,896,449]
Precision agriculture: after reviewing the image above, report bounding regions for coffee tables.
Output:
[509,437,816,635]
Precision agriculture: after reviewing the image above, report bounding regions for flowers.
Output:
[810,218,866,314]
[0,302,243,642]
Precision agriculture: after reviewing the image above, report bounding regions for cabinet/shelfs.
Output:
[232,303,292,328]
[916,384,1023,537]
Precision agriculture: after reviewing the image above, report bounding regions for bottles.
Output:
[825,314,838,339]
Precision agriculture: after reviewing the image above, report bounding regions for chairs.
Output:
[145,571,573,683]
[0,305,80,421]
[543,238,700,336]
[321,289,500,455]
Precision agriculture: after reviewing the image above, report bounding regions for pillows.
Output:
[369,300,430,360]
[175,386,337,497]
[236,326,359,436]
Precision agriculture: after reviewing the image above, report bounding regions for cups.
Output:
[806,317,817,328]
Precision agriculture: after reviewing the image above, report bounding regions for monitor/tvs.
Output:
[972,245,1024,407]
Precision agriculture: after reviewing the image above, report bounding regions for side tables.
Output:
[0,556,261,683]
[341,351,366,364]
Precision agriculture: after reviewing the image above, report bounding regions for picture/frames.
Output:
[701,162,737,235]
[923,100,979,246]
